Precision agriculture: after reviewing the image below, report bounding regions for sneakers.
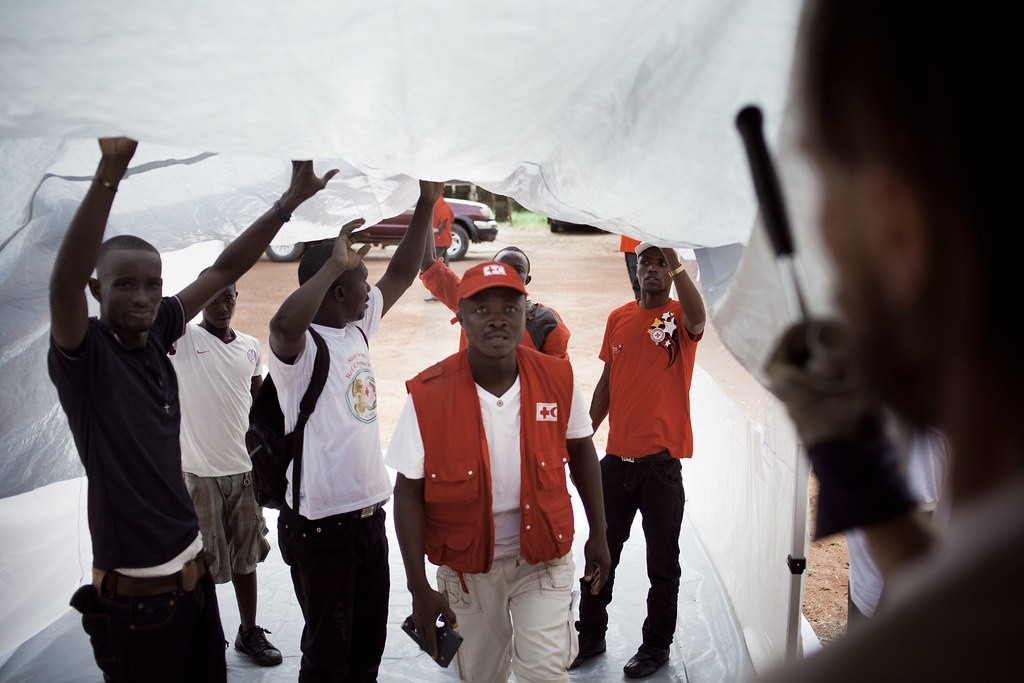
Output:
[566,634,606,670]
[235,625,282,667]
[624,647,670,678]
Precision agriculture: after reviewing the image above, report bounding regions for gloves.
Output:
[762,319,923,542]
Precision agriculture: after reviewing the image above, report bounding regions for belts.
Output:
[92,547,217,597]
[619,451,671,463]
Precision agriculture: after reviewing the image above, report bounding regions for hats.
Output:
[449,261,527,325]
[635,242,680,262]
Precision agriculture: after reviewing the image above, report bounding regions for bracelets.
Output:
[438,228,441,231]
[274,201,292,223]
[92,176,119,193]
[668,264,686,278]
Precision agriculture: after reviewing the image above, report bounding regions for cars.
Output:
[264,197,499,262]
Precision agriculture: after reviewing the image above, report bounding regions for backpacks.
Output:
[246,324,370,509]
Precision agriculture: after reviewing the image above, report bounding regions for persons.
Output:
[418,211,573,359]
[266,180,444,683]
[568,242,706,678]
[620,234,642,300]
[423,196,455,301]
[167,267,283,667]
[47,135,339,683]
[384,260,612,683]
[745,1,1024,683]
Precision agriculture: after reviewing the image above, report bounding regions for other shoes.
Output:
[424,295,436,301]
